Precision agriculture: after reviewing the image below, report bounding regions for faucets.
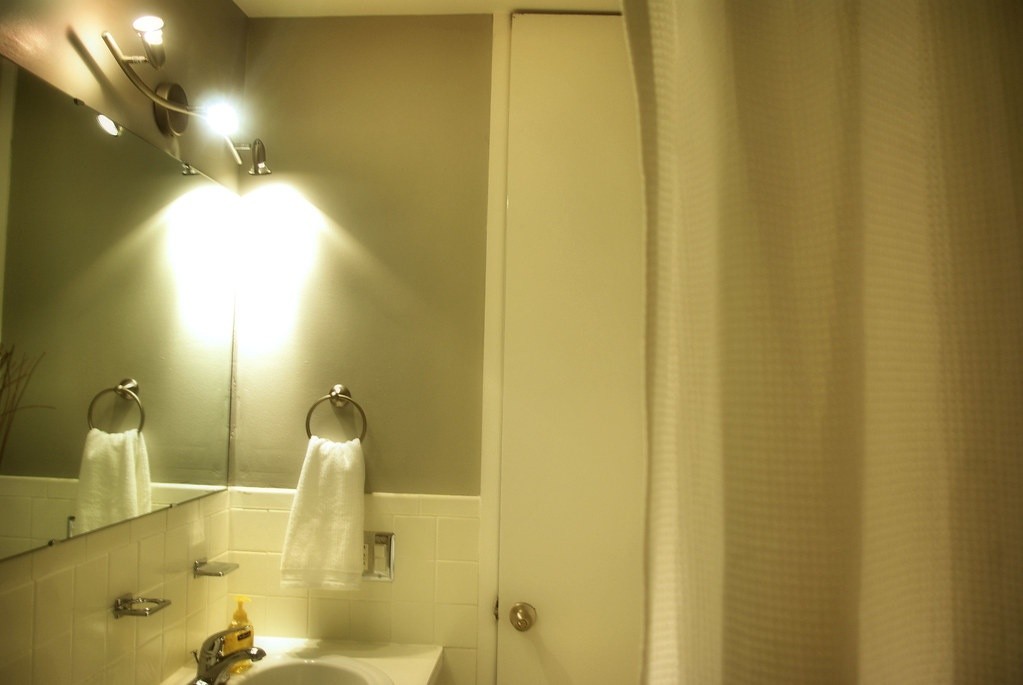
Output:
[187,625,266,685]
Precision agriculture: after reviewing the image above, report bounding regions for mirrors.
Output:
[0,54,240,561]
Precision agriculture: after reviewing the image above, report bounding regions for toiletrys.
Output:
[222,593,256,674]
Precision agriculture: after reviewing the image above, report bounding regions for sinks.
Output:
[225,651,393,684]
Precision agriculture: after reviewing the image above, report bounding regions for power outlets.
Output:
[362,531,395,581]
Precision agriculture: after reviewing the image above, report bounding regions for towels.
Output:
[76,429,152,537]
[278,436,366,590]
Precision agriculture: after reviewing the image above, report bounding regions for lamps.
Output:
[102,16,274,177]
